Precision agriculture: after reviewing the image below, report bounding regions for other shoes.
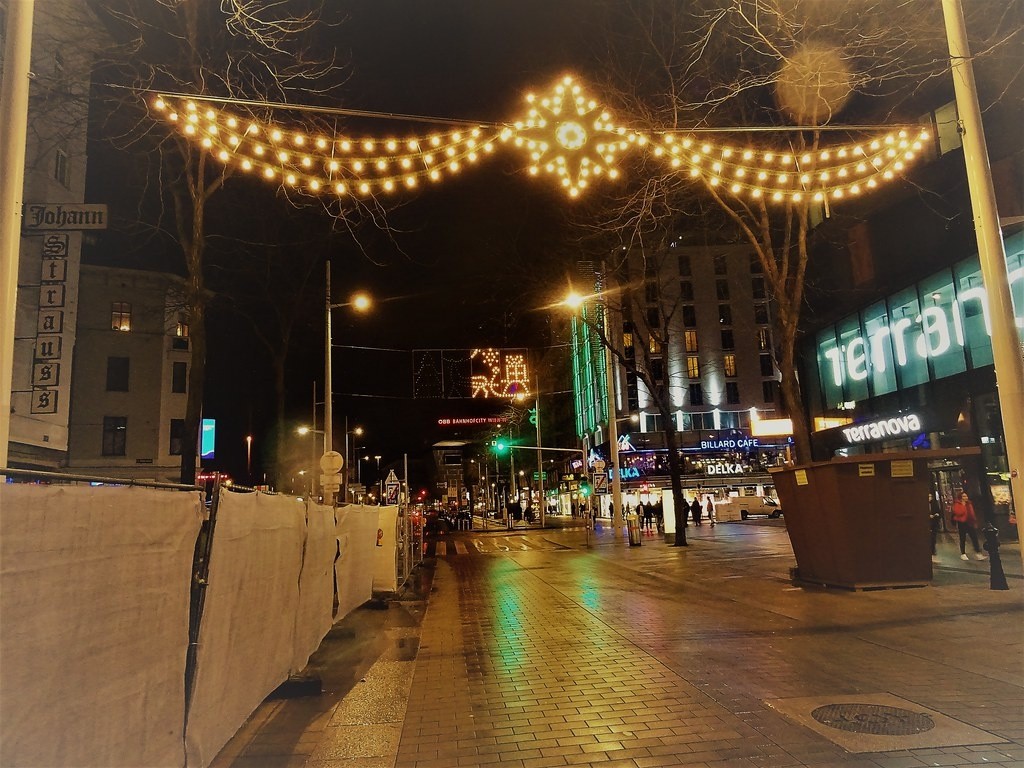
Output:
[710,522,714,529]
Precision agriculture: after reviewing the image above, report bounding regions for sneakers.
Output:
[974,553,988,561]
[960,553,969,561]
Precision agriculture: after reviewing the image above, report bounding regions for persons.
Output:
[953,491,988,561]
[571,502,598,524]
[1008,477,1020,543]
[929,493,942,555]
[608,497,716,533]
[548,504,557,516]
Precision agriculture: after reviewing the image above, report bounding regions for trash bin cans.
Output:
[507,513,514,530]
[626,515,641,547]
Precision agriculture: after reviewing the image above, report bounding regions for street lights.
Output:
[297,427,325,456]
[566,294,623,540]
[345,428,364,503]
[517,394,544,527]
[322,294,372,505]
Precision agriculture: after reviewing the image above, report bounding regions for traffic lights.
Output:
[497,443,505,452]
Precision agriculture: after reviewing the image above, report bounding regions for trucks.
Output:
[731,496,782,520]
